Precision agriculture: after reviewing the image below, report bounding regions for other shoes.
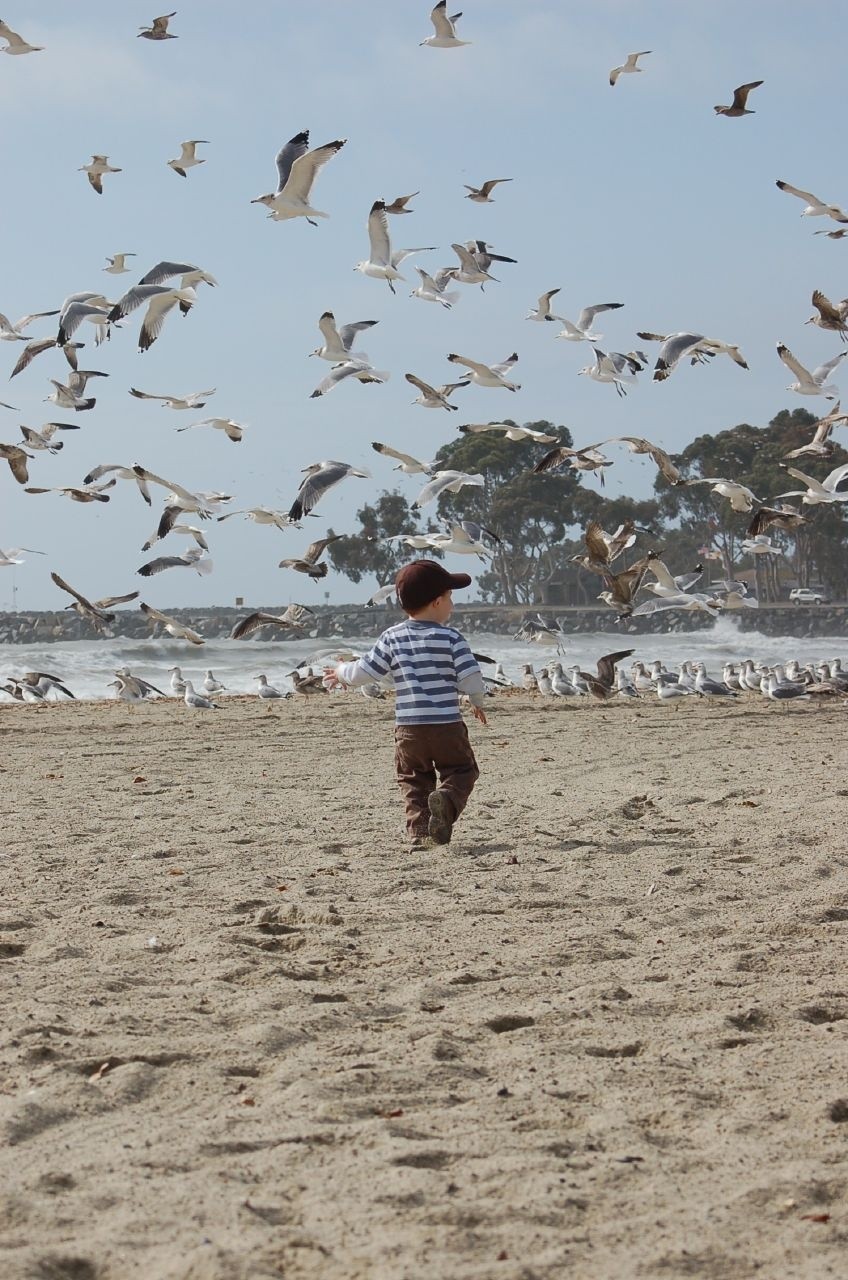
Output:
[428,791,454,844]
[410,836,425,845]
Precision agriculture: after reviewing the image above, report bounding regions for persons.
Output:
[323,560,492,845]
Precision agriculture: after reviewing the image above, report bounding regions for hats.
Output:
[396,559,471,607]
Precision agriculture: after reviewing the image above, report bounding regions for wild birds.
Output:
[136,11,180,40]
[814,228,848,238]
[713,80,764,116]
[775,179,847,223]
[748,289,848,703]
[609,50,653,85]
[419,0,472,49]
[2,131,760,708]
[0,21,47,54]
